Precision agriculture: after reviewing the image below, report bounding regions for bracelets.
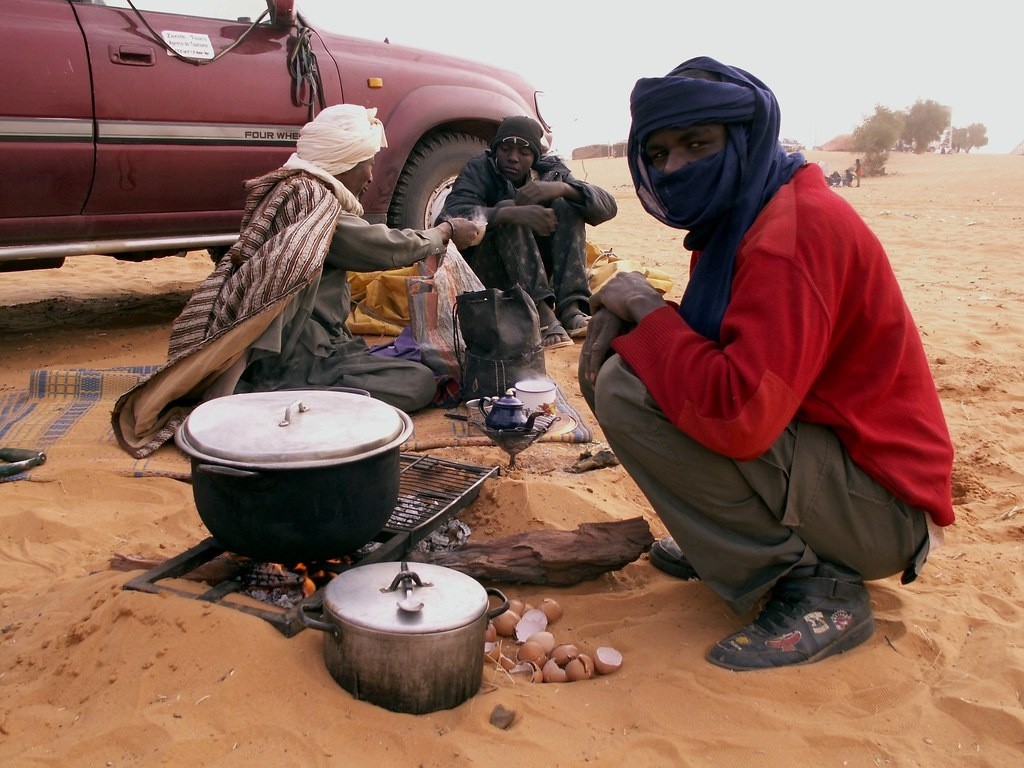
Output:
[443,220,454,239]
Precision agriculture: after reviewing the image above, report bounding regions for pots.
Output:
[174,386,415,563]
[298,562,510,716]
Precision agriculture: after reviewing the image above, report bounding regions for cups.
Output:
[507,380,558,413]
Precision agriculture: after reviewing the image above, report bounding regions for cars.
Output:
[927,141,954,153]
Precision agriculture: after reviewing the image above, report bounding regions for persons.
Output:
[440,117,616,350]
[580,56,955,669]
[816,159,861,188]
[109,105,479,459]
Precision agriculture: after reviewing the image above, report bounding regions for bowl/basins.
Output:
[443,399,494,436]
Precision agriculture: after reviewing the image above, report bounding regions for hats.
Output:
[293,102,388,175]
[490,114,544,167]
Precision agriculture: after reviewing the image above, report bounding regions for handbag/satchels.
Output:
[454,282,547,399]
[406,239,487,383]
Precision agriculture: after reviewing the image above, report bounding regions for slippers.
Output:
[562,311,594,337]
[540,319,574,350]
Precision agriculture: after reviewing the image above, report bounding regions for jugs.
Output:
[479,390,545,431]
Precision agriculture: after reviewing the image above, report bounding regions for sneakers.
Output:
[649,538,700,581]
[707,563,875,672]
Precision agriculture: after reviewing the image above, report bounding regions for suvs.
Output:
[1,1,554,272]
